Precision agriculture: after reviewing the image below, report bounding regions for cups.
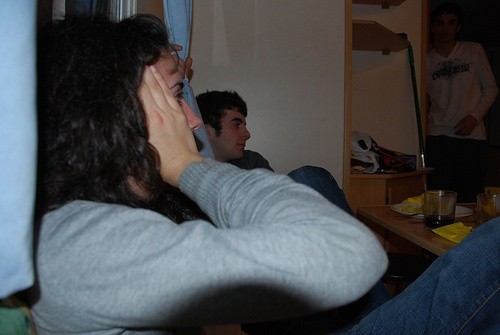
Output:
[422,190,457,230]
[475,186,499,228]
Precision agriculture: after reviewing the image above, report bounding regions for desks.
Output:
[358,201,492,257]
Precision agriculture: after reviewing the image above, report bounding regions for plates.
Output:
[391,200,474,219]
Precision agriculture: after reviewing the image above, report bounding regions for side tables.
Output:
[346,19,435,213]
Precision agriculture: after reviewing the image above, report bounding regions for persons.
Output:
[419,0,499,205]
[194,89,390,335]
[29,11,500,335]
